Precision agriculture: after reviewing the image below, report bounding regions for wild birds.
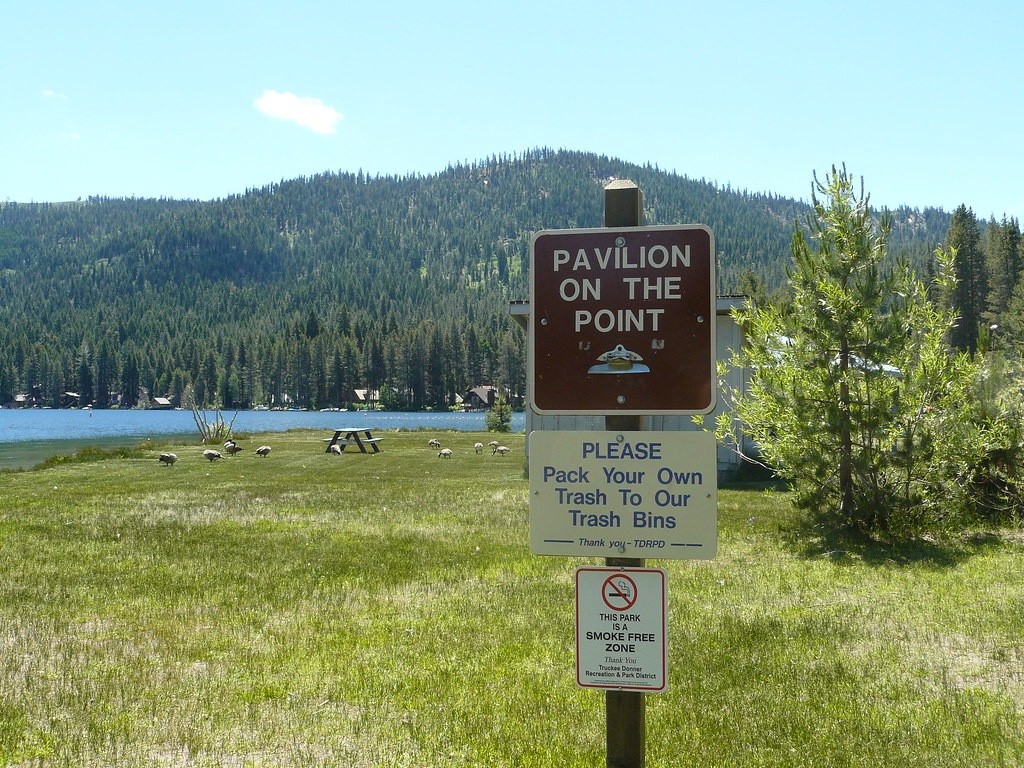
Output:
[428,438,512,458]
[253,446,271,458]
[158,452,181,466]
[203,439,245,462]
[330,444,342,455]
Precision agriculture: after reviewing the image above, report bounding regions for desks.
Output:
[325,427,379,454]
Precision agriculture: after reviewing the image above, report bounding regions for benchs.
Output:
[322,436,345,443]
[362,438,385,443]
